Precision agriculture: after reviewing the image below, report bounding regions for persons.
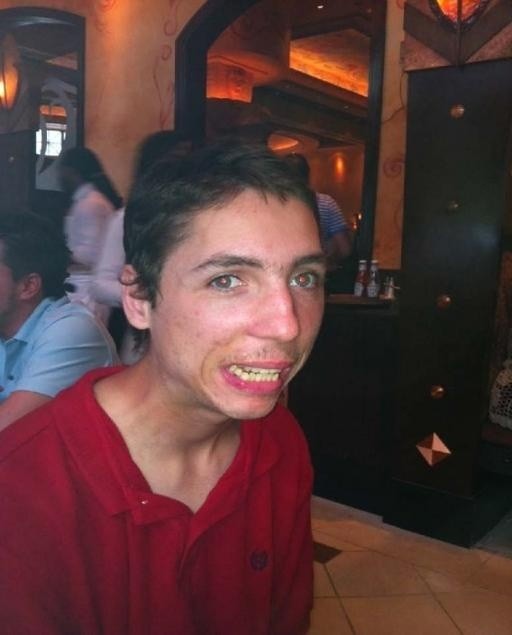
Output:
[58,147,124,351]
[0,136,327,635]
[0,206,124,426]
[93,129,184,364]
[282,153,351,296]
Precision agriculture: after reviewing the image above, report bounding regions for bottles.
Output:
[354,260,368,297]
[367,260,381,296]
